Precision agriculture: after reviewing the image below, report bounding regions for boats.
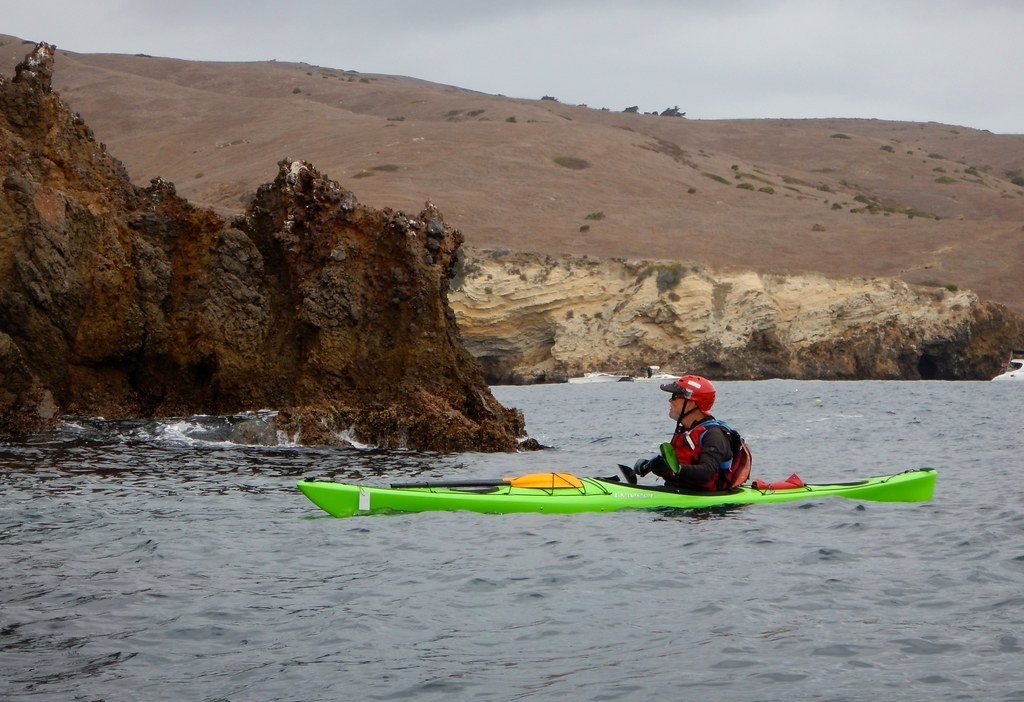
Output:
[296,468,938,517]
[568,365,681,380]
[991,356,1024,381]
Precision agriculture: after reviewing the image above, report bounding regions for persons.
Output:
[634,375,738,496]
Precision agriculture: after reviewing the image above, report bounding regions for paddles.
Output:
[618,442,679,485]
[389,473,583,489]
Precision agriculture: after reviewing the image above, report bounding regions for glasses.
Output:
[672,393,685,400]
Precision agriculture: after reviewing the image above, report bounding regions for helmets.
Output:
[660,375,716,413]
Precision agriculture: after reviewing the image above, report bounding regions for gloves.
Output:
[649,455,673,479]
[634,459,652,477]
